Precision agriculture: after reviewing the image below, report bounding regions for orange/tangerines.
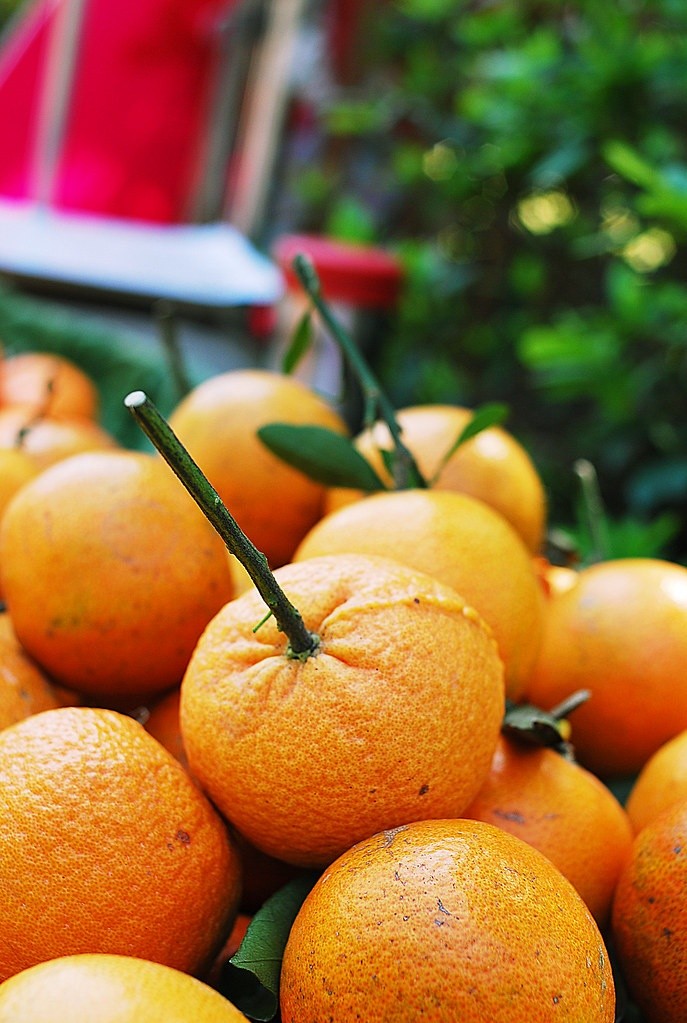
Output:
[0,353,687,1023]
